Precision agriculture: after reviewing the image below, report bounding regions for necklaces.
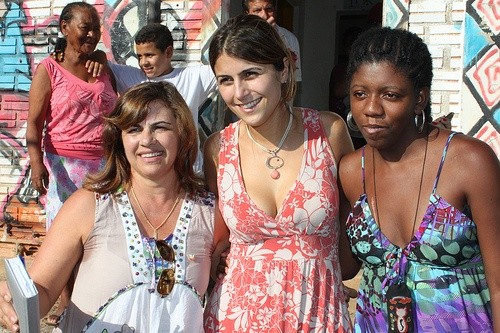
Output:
[131,183,183,239]
[245,106,293,179]
[371,125,431,283]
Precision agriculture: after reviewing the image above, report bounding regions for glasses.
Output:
[154,239,175,298]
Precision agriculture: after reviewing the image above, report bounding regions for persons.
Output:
[242,0,302,106]
[200,15,360,333]
[0,82,216,333]
[25,2,119,234]
[85,17,275,178]
[336,27,500,333]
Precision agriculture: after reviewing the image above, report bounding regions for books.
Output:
[5,255,41,333]
